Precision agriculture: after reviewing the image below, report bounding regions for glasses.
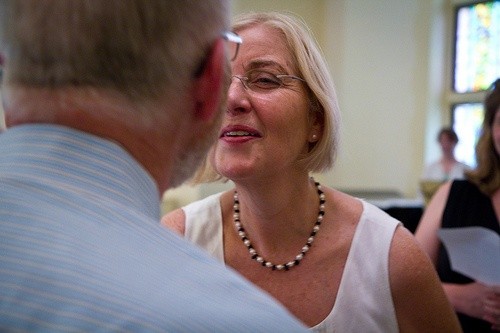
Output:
[231,68,304,94]
[194,30,243,79]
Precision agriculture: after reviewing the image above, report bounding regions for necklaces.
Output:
[233,177,326,273]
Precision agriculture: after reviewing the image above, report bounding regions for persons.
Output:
[0,0,318,333]
[414,77,500,333]
[160,10,464,333]
[420,128,472,208]
